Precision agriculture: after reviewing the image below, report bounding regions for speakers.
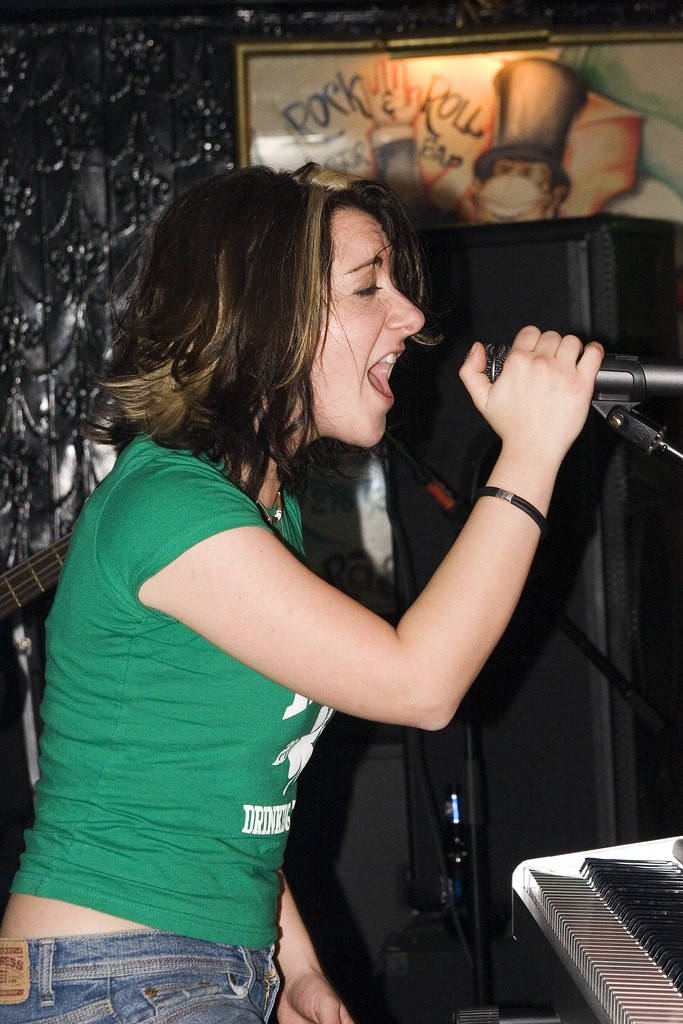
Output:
[382,215,683,914]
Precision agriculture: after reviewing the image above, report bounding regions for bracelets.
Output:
[479,486,546,532]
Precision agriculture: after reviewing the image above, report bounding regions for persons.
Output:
[0,161,607,1024]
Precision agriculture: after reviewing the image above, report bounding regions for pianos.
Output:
[505,831,683,1024]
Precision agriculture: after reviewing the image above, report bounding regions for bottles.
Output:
[443,790,472,916]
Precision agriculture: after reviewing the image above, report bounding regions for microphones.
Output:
[481,343,683,400]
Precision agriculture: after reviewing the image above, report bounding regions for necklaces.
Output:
[222,455,283,524]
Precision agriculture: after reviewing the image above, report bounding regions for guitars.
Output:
[0,529,75,621]
